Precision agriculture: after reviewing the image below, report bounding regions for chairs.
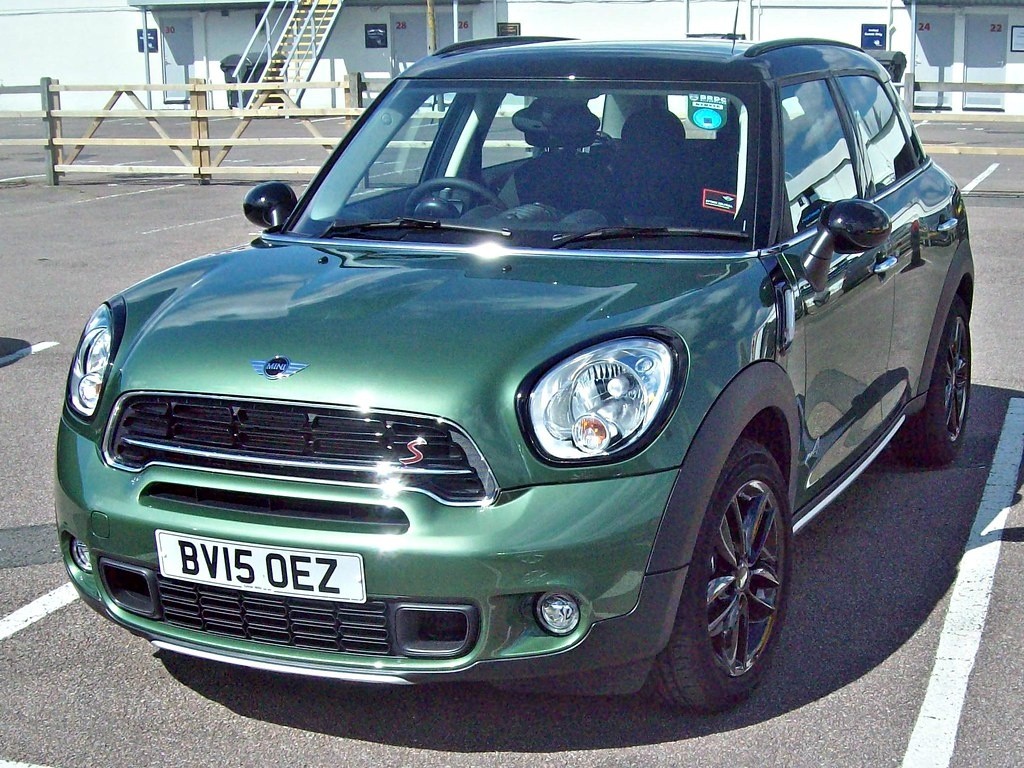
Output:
[498,99,610,215]
[620,109,703,219]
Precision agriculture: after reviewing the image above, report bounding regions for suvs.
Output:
[54,37,978,709]
